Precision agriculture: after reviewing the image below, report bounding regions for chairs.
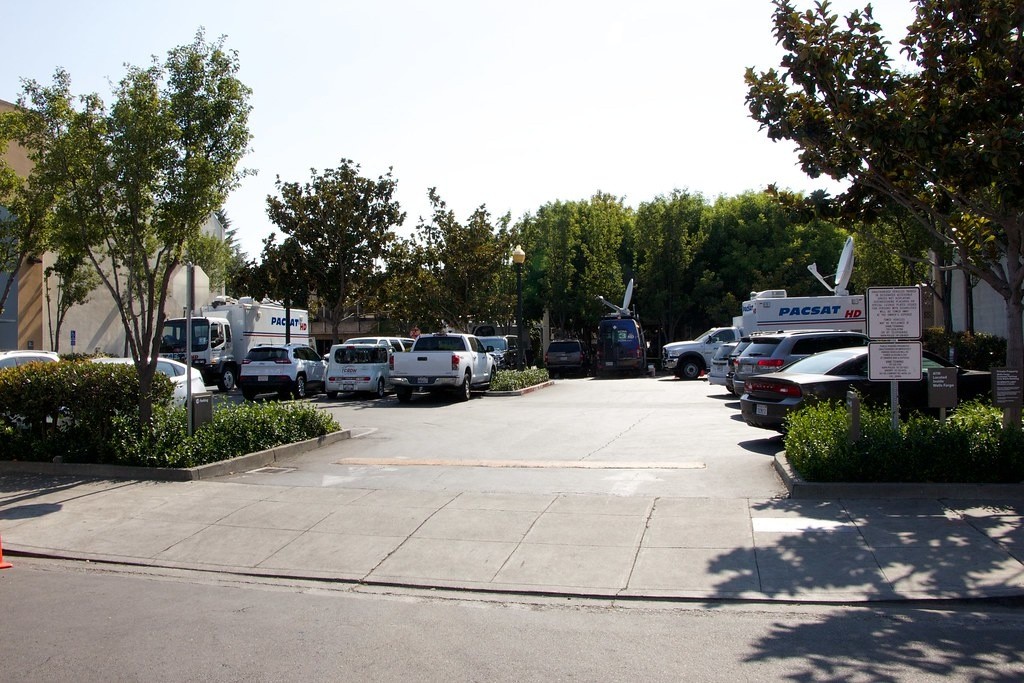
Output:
[207,330,222,344]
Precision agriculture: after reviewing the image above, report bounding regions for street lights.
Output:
[513,244,526,371]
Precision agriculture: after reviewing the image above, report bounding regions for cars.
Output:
[738,346,993,434]
[81,358,207,420]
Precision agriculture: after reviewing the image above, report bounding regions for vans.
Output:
[326,344,397,399]
[323,337,417,378]
[597,319,648,378]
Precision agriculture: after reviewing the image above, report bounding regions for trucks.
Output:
[660,289,868,381]
[158,296,310,393]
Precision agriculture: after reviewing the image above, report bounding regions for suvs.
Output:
[240,342,329,401]
[708,341,737,392]
[732,329,871,396]
[726,331,775,396]
[543,338,595,378]
[0,351,64,376]
[476,335,518,363]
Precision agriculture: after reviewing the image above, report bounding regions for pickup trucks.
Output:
[389,330,498,404]
